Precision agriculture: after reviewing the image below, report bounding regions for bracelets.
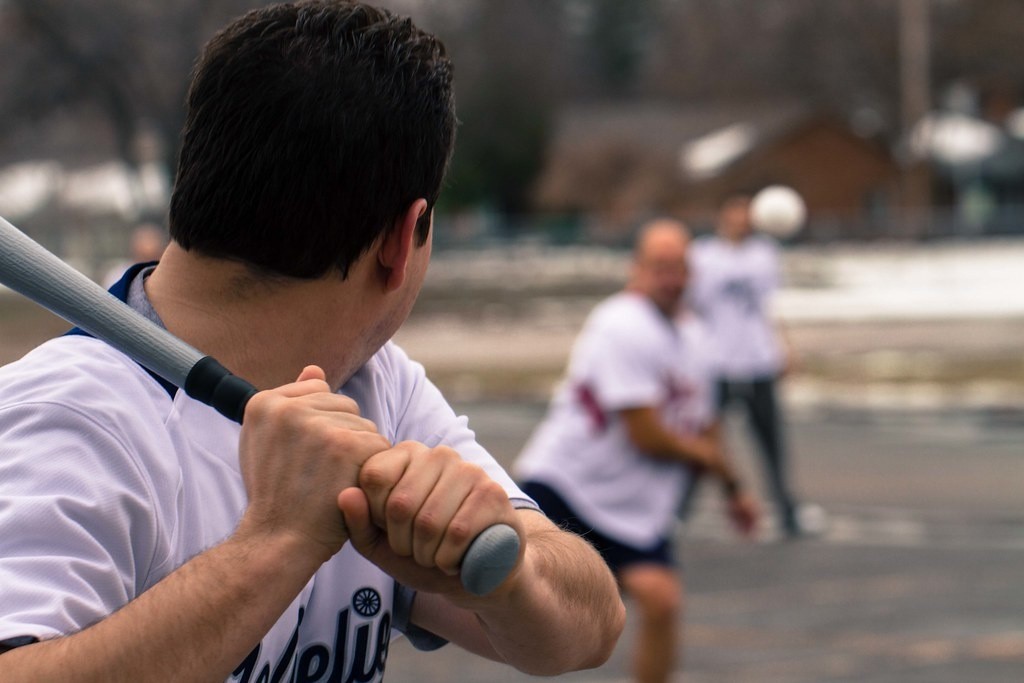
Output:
[719,476,739,495]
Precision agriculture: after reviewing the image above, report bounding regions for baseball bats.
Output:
[0,216,522,595]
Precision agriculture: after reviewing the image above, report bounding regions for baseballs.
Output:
[748,183,808,239]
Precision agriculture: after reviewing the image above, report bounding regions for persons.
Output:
[676,191,827,538]
[0,0,628,683]
[510,214,772,682]
